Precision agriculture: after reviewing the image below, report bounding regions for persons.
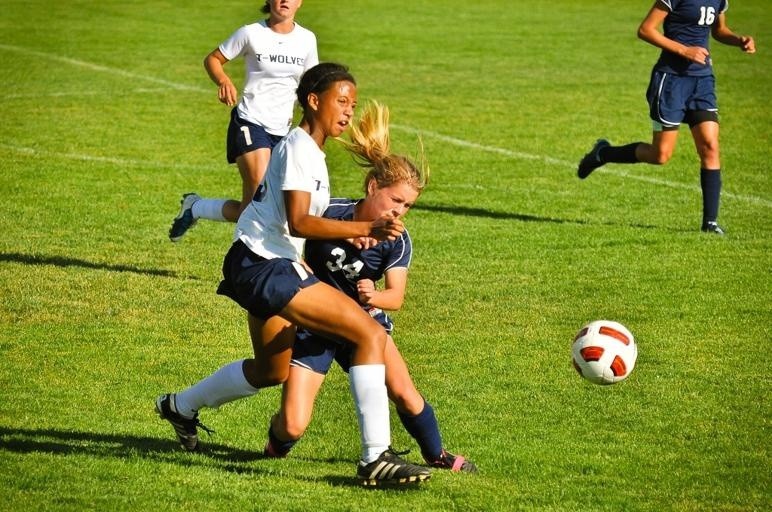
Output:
[153,63,433,487]
[576,1,756,234]
[166,0,319,245]
[265,96,480,479]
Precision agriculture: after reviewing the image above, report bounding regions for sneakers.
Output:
[265,442,290,456]
[153,393,199,452]
[423,447,476,472]
[701,221,724,233]
[578,139,609,178]
[357,445,430,485]
[169,193,202,242]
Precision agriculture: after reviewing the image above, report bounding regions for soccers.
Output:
[572,320,637,384]
